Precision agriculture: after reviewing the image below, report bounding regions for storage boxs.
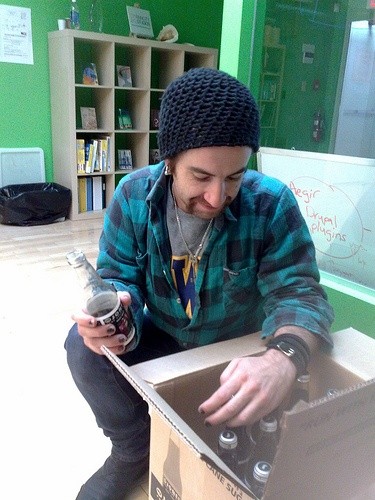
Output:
[100,326,375,500]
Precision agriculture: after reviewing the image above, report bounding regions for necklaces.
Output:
[172,194,214,265]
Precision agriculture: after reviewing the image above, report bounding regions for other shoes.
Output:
[76,451,149,500]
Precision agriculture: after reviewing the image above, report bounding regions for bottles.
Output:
[65,249,140,355]
[215,369,338,498]
[70,0,80,29]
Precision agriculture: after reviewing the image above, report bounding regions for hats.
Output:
[157,67,260,160]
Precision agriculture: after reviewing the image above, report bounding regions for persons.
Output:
[62,68,333,499]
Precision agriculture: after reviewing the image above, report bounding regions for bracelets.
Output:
[264,333,311,378]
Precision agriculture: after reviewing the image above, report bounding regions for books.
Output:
[79,138,114,217]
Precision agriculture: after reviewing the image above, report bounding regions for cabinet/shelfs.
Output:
[250,43,286,171]
[48,29,218,222]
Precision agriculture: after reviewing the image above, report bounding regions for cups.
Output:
[57,19,66,30]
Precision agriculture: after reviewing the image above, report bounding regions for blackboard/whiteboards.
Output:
[255,145,374,308]
